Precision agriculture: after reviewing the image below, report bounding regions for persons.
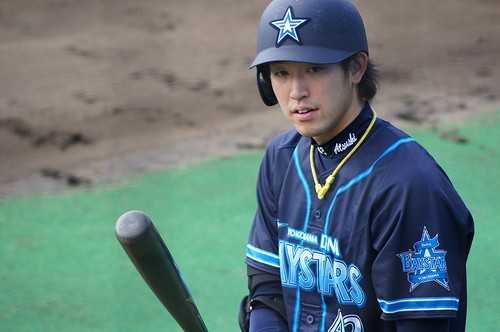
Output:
[235,0,477,331]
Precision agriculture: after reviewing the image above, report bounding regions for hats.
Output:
[248,0,369,71]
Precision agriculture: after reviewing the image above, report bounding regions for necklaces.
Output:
[308,101,378,201]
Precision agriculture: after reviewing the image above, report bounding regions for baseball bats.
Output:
[115,210,210,332]
